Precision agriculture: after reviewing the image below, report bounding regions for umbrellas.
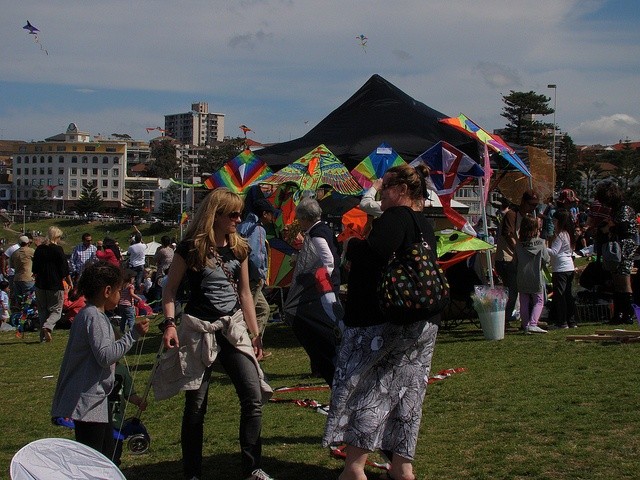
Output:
[496,146,556,205]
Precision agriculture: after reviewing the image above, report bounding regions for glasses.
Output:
[61,237,64,240]
[86,239,92,242]
[382,182,399,189]
[528,201,541,209]
[229,211,243,219]
[117,244,120,246]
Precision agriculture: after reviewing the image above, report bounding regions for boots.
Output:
[608,291,622,325]
[617,292,633,324]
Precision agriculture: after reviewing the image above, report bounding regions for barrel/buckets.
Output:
[479,310,505,340]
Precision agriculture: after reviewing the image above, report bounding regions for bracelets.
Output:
[158,317,176,332]
[162,323,176,333]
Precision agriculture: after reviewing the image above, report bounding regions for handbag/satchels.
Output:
[579,261,612,290]
[376,205,453,312]
[601,230,622,273]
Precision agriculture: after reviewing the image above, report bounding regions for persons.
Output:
[153,235,175,280]
[495,190,540,332]
[322,165,441,480]
[127,231,147,294]
[96,237,123,272]
[583,203,591,216]
[545,209,578,330]
[569,197,580,225]
[32,226,73,343]
[0,281,11,326]
[119,269,142,335]
[177,211,182,225]
[578,206,588,224]
[474,216,494,247]
[62,272,87,326]
[515,215,551,335]
[570,225,586,254]
[129,224,143,245]
[0,238,8,280]
[2,233,34,290]
[540,189,580,248]
[143,267,153,296]
[286,197,345,391]
[595,182,639,324]
[10,235,38,332]
[113,240,123,263]
[70,233,98,282]
[234,197,277,362]
[161,186,274,480]
[50,261,151,468]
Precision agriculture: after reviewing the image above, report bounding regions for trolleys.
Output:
[52,336,175,454]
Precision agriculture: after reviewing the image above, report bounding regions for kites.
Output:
[433,228,495,273]
[45,185,58,191]
[265,194,303,288]
[258,144,366,206]
[203,146,275,201]
[146,126,172,135]
[336,200,373,244]
[6,283,39,333]
[181,212,188,224]
[149,133,177,143]
[356,34,368,55]
[438,112,536,179]
[282,233,346,346]
[22,20,48,55]
[239,124,256,141]
[319,140,413,218]
[407,140,486,240]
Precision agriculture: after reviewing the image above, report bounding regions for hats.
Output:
[103,237,115,244]
[251,198,274,213]
[18,236,30,244]
[556,188,580,205]
[24,233,34,239]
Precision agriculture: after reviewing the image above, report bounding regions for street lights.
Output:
[547,85,556,206]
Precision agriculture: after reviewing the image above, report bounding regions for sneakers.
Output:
[249,469,273,480]
[528,326,548,333]
